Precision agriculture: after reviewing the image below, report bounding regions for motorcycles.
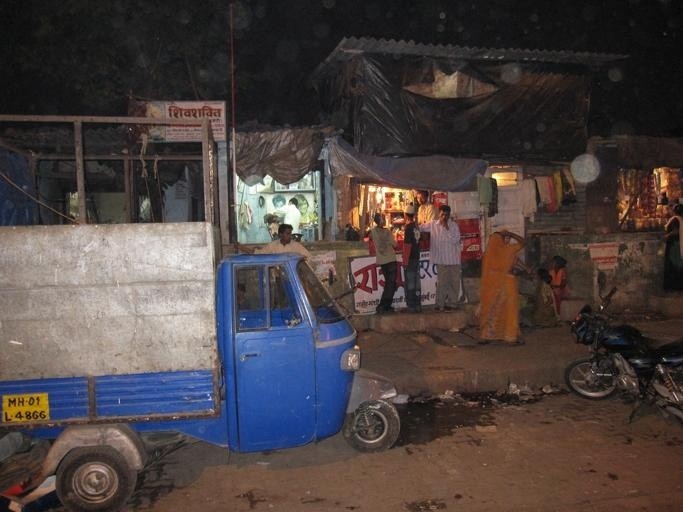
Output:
[564,269,682,425]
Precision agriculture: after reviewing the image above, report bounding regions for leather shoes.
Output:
[433,305,458,311]
[376,305,407,313]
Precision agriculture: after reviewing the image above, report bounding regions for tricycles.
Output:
[2,252,400,512]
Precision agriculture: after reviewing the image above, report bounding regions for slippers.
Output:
[477,338,524,345]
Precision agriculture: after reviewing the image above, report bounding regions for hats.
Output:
[405,206,416,213]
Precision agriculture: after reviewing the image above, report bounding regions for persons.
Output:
[274,197,303,239]
[371,189,569,346]
[239,223,312,310]
[661,202,682,294]
